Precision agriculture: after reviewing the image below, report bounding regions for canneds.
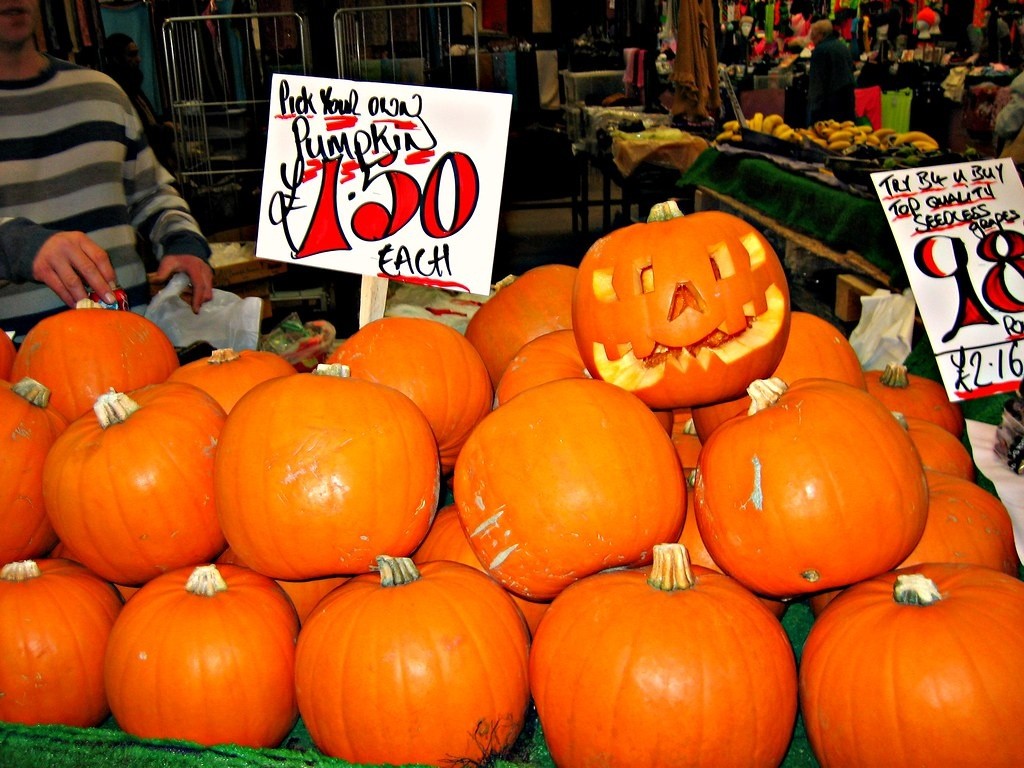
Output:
[89,287,128,311]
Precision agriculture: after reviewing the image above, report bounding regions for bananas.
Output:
[714,113,938,151]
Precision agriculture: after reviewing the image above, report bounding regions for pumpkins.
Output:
[0,265,1024,768]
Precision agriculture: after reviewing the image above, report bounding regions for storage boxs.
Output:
[146,226,286,320]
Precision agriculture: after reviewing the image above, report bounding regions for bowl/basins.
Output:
[738,127,911,187]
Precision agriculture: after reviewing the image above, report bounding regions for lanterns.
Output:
[573,200,791,406]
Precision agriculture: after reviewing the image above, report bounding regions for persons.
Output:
[762,30,781,58]
[916,8,942,38]
[995,72,1024,170]
[103,32,205,170]
[0,0,215,365]
[964,18,1013,159]
[809,19,854,125]
[781,15,810,57]
[740,17,755,37]
[895,29,908,50]
[875,15,889,40]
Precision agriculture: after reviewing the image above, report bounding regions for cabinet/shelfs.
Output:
[696,185,927,342]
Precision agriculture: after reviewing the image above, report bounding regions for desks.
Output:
[572,122,695,260]
